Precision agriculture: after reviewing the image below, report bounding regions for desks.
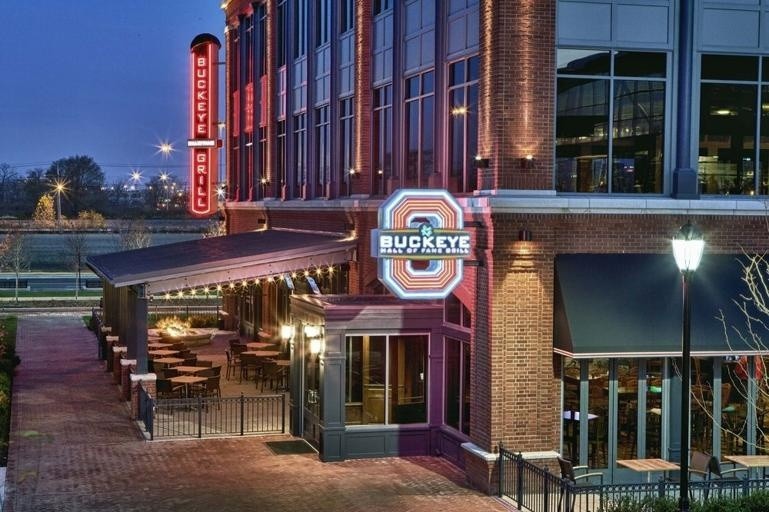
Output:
[562,385,713,469]
[724,455,769,498]
[614,458,680,508]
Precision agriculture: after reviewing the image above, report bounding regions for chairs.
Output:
[701,382,766,455]
[669,450,749,511]
[556,454,604,512]
[146,327,289,416]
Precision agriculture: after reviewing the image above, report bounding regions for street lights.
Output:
[57,185,63,220]
[161,144,169,210]
[670,220,706,510]
